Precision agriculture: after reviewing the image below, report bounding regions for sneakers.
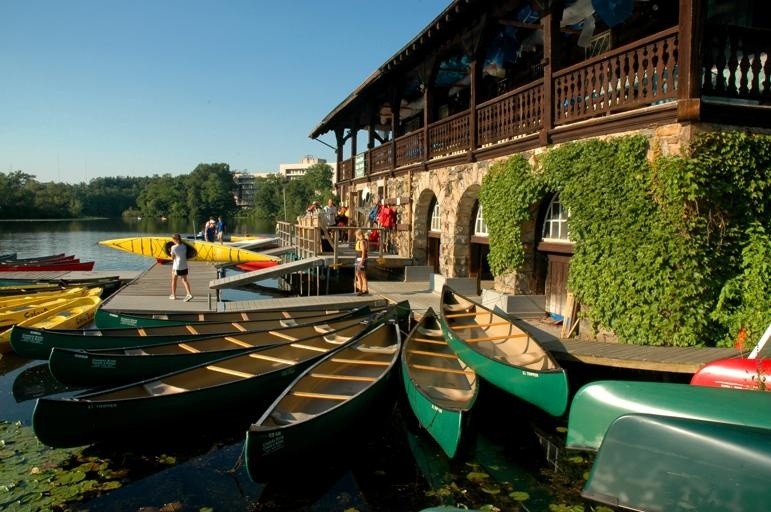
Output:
[183,293,193,302]
[169,295,176,300]
[358,290,369,296]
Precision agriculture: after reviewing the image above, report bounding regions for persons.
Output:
[355,228,368,296]
[169,233,193,302]
[306,198,338,235]
[204,215,226,245]
